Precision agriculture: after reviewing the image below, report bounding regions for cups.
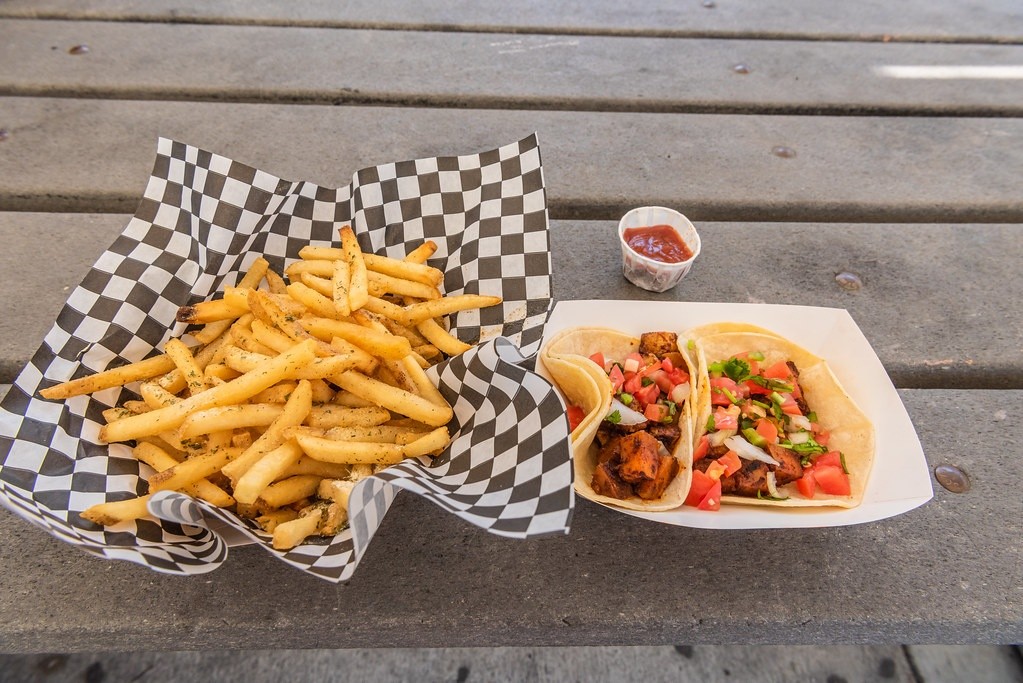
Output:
[618,207,702,292]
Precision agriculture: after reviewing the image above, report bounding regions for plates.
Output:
[531,299,935,531]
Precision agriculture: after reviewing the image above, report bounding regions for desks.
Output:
[0,0,1023,648]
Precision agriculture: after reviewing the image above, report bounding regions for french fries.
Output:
[37,225,504,552]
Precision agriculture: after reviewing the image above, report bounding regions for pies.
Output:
[540,320,876,511]
